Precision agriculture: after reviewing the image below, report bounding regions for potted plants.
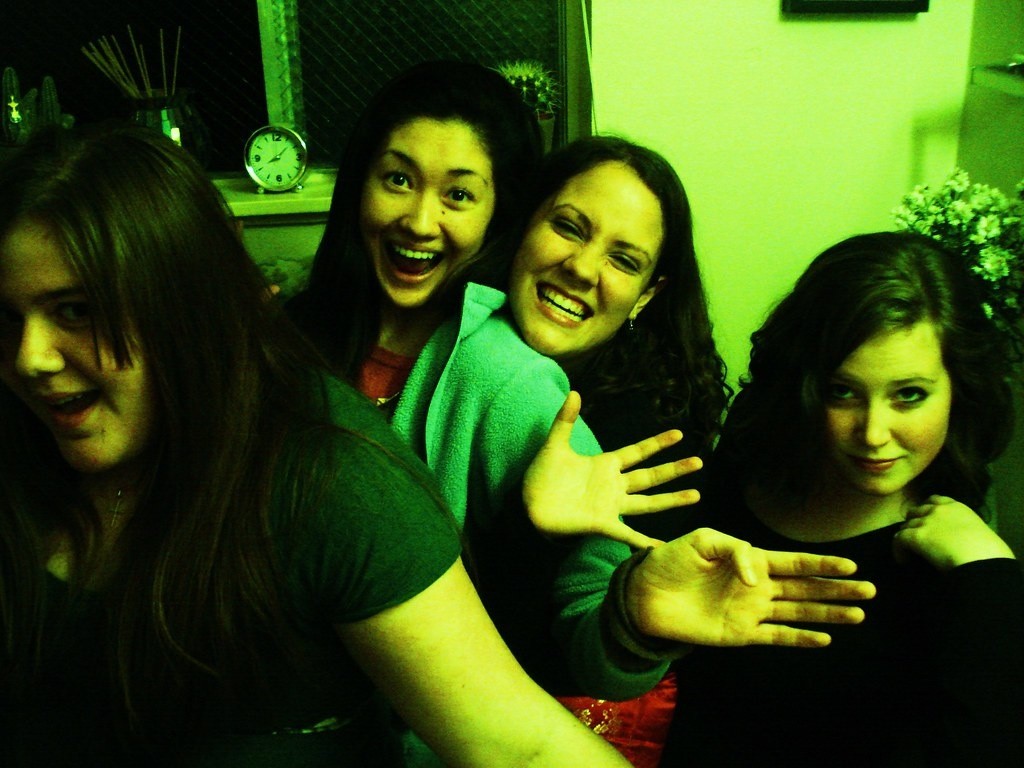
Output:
[492,59,565,154]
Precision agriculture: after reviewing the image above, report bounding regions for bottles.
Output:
[125,87,215,173]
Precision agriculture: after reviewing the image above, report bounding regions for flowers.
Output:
[892,164,1024,359]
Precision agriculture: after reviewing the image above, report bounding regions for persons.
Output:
[674,229,1024,768]
[281,59,875,768]
[502,135,736,767]
[0,125,633,768]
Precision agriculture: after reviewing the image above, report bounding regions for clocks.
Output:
[243,122,307,193]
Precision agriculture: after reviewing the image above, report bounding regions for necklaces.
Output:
[105,481,130,528]
